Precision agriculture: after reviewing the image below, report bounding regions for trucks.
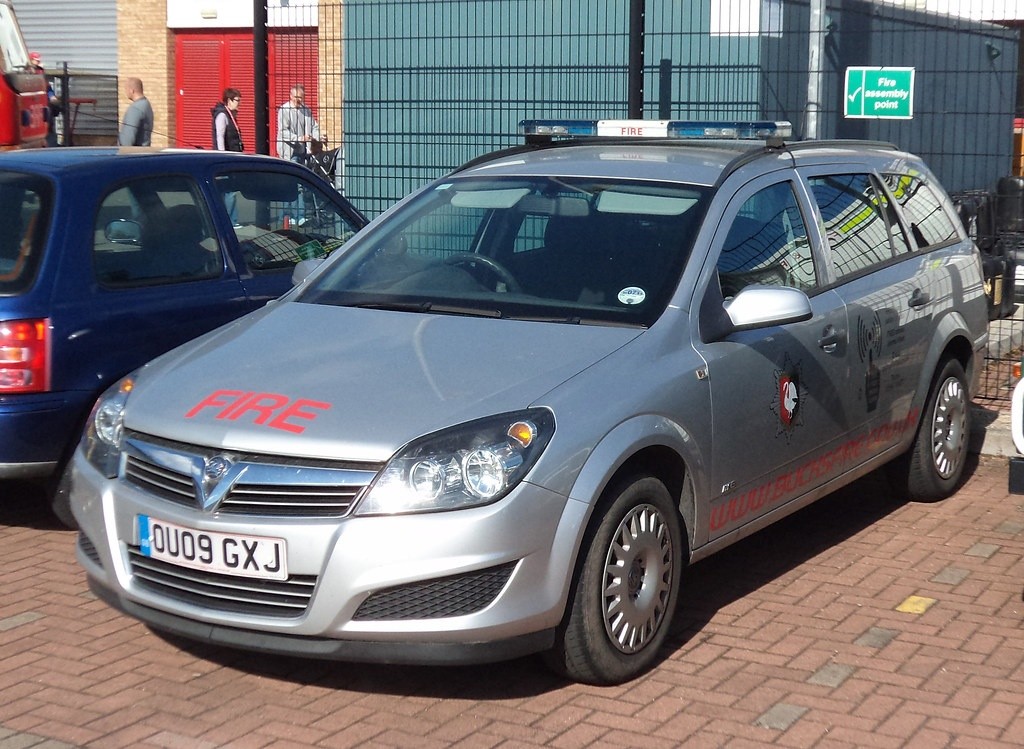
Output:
[0,0,61,150]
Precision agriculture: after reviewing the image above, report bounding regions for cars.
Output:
[63,117,990,686]
[1,145,440,534]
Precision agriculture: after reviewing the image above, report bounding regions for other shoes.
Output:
[232,223,243,229]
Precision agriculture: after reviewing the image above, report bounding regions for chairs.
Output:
[528,213,633,304]
[166,204,214,277]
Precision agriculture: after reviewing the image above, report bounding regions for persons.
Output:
[118,78,154,146]
[28,52,57,101]
[277,84,328,162]
[211,87,246,151]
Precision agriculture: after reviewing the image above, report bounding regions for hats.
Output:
[29,51,41,61]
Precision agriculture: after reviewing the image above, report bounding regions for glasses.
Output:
[233,99,241,102]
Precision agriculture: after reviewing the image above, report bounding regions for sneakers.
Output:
[288,216,309,227]
